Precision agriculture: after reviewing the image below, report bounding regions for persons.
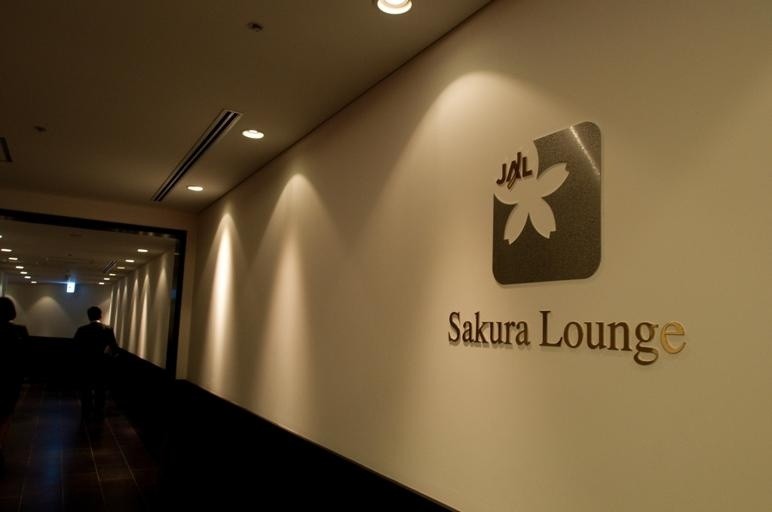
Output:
[67,306,118,431]
[0,296,33,450]
